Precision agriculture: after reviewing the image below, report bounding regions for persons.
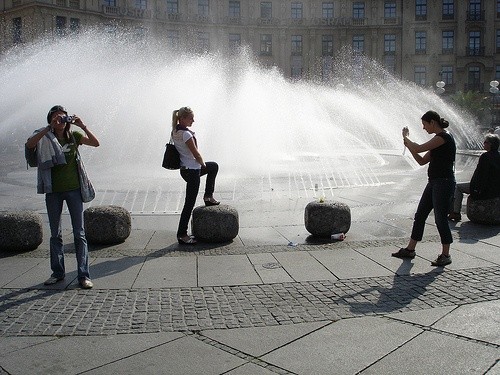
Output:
[391,110,456,265]
[24,105,100,289]
[448,134,500,222]
[168,106,221,245]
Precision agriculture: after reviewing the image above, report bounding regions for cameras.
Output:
[61,115,72,123]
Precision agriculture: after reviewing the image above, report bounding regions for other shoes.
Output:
[80,279,93,288]
[448,212,462,220]
[431,254,452,266]
[392,248,415,258]
[45,276,63,284]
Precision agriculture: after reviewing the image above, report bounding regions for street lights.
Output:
[489,75,499,131]
[434,71,446,99]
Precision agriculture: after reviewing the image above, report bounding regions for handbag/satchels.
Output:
[162,138,180,170]
[77,155,95,202]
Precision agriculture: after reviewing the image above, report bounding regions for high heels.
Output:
[204,197,220,206]
[178,236,196,244]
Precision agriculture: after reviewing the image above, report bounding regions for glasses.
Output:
[484,141,489,144]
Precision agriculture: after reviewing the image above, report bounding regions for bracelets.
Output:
[83,126,86,130]
[202,162,206,166]
[48,126,52,129]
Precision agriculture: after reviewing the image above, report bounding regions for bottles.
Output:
[331,233,344,240]
